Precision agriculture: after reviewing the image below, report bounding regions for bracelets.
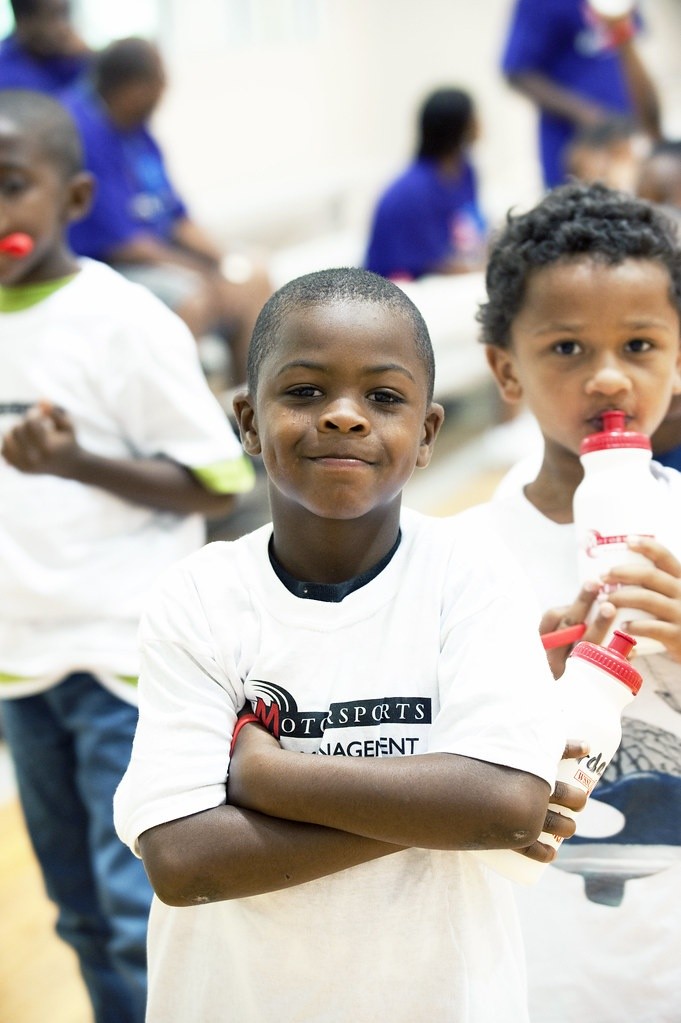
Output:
[228,713,259,751]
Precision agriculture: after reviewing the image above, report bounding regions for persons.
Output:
[502,0,680,204]
[1,0,97,93]
[111,268,586,1023]
[2,88,258,1023]
[65,36,273,385]
[363,87,488,281]
[443,193,680,1023]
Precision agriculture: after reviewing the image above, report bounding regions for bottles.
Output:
[537,628,645,852]
[572,410,667,657]
